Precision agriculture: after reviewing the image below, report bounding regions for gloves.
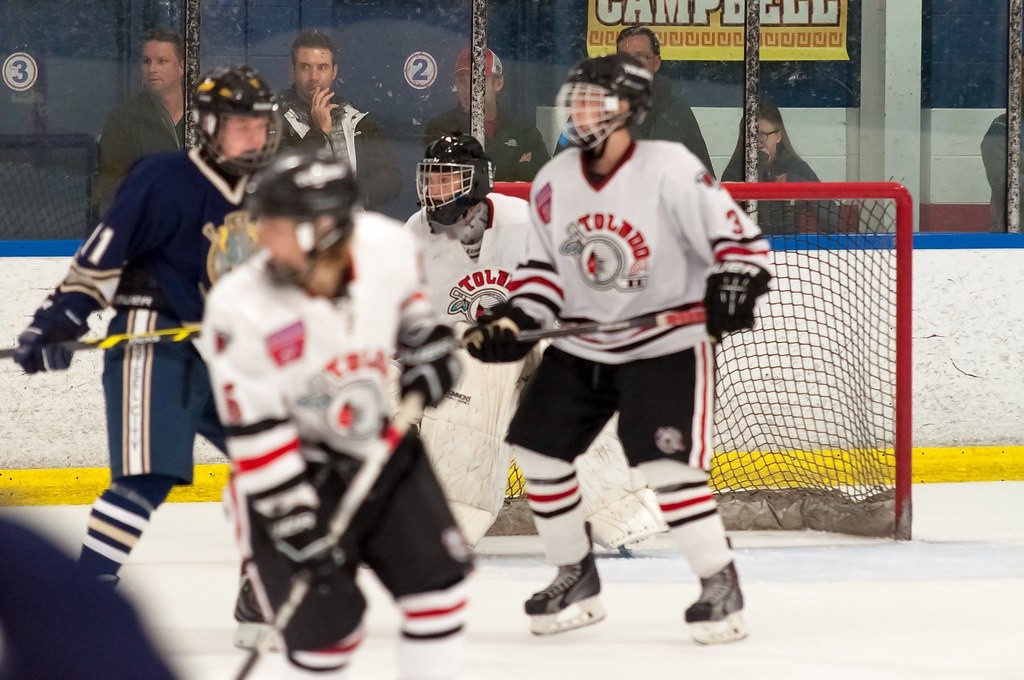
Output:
[703,263,771,343]
[248,474,349,582]
[467,304,540,363]
[394,330,463,403]
[9,287,103,375]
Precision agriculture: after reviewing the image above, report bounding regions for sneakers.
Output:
[684,563,752,648]
[524,553,609,638]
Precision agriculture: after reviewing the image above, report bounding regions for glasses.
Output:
[754,128,781,143]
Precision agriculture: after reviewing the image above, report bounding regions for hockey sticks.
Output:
[397,307,707,366]
[232,390,426,679]
[0,325,201,358]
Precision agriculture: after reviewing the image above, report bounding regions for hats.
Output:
[451,46,503,79]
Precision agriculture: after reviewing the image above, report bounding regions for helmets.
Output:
[240,151,357,254]
[556,55,654,147]
[190,66,281,169]
[412,135,492,226]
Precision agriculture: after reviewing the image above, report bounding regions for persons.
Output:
[98,27,207,221]
[721,103,838,235]
[275,32,404,214]
[405,129,536,553]
[979,110,1008,233]
[15,66,281,627]
[424,46,551,182]
[552,26,717,179]
[502,50,772,622]
[200,151,482,680]
